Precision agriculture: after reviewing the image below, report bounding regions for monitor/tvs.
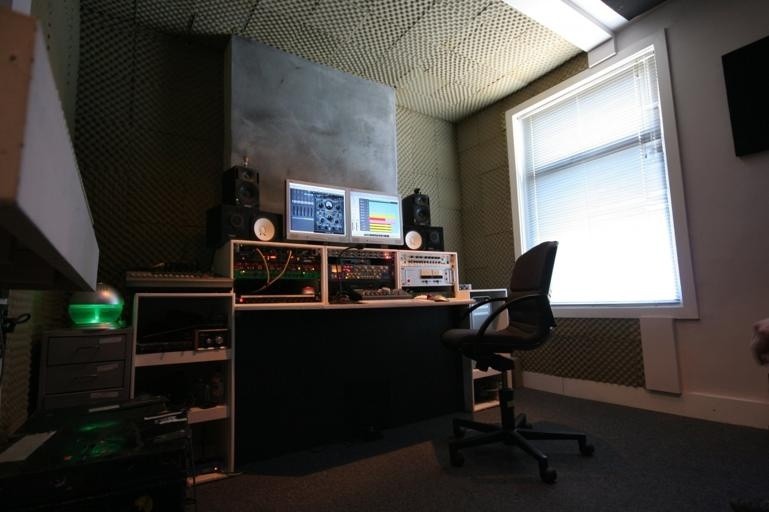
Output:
[285,178,348,243]
[348,187,403,246]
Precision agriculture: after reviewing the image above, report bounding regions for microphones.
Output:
[335,243,365,302]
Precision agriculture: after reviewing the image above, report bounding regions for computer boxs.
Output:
[471,295,492,329]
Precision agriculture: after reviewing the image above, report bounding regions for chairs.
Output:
[443,241,594,484]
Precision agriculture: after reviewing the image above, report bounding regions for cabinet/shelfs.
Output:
[130,293,235,485]
[29,328,133,434]
[455,289,512,412]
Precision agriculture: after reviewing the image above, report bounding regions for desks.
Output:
[235,298,475,455]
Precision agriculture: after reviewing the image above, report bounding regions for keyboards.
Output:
[345,288,413,299]
[124,271,232,288]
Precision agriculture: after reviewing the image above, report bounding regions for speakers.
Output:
[388,227,444,252]
[204,208,282,247]
[402,194,430,227]
[221,164,259,211]
